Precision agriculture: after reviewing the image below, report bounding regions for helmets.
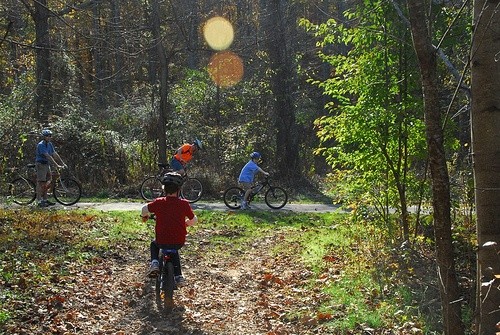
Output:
[42,130,53,136]
[193,137,202,150]
[250,152,260,159]
[161,172,184,185]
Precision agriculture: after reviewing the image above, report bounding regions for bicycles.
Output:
[223,173,288,209]
[139,214,192,313]
[9,164,83,206]
[141,163,204,204]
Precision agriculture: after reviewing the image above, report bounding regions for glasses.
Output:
[46,135,51,137]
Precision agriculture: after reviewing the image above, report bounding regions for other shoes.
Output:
[38,200,56,207]
[146,262,160,276]
[241,202,251,211]
[175,275,184,284]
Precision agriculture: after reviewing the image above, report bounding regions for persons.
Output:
[240,152,270,210]
[142,172,198,289]
[36,130,68,207]
[173,139,202,175]
